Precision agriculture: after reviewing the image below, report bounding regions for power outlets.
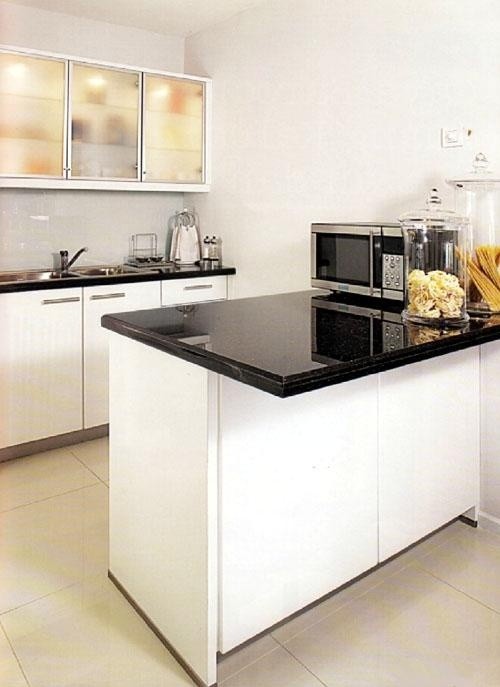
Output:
[441,127,463,147]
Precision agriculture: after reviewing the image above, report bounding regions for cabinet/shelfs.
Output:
[102,291,500,687]
[69,57,141,190]
[142,69,212,191]
[0,46,69,188]
[0,280,160,452]
[162,274,229,306]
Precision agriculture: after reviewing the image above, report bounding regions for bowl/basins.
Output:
[136,258,148,264]
[150,257,163,262]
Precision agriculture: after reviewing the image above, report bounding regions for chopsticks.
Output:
[466,247,500,312]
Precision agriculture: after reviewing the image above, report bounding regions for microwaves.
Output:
[310,223,416,302]
